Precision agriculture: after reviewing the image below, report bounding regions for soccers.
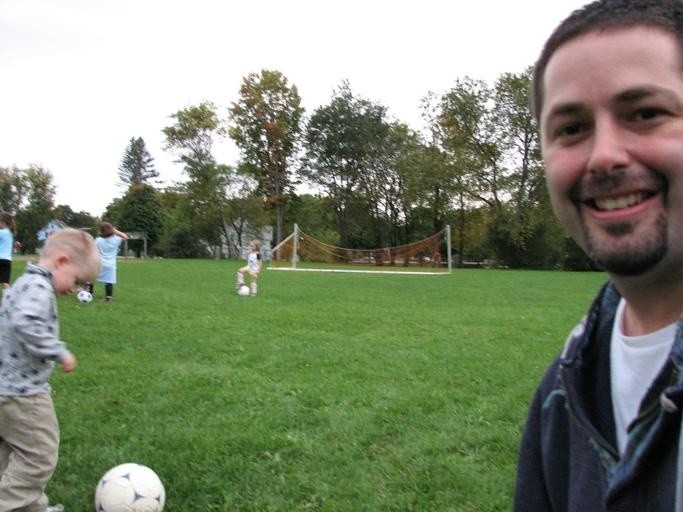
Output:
[93,462,165,511]
[237,285,251,298]
[76,289,93,304]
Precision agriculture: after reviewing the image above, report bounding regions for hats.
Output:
[100,222,112,235]
[251,239,260,251]
[0,214,12,223]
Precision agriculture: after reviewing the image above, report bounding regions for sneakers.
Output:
[45,504,65,512]
[236,282,245,288]
[250,292,257,296]
[105,296,112,301]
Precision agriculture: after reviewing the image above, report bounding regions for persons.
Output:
[0,226,103,511]
[84,222,128,302]
[0,210,17,288]
[506,0,683,511]
[238,239,263,297]
